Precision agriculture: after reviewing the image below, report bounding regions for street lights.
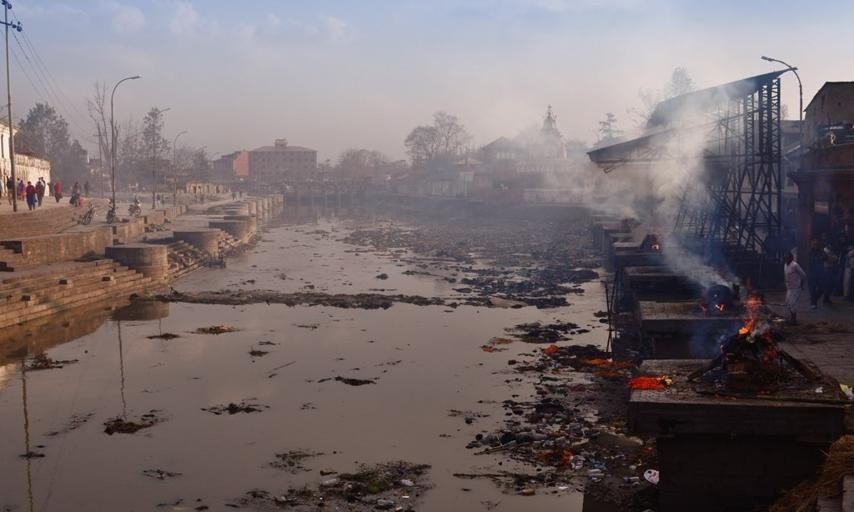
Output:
[151,107,188,208]
[761,55,804,169]
[110,74,142,209]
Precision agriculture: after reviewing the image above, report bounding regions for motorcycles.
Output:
[106,205,119,224]
[125,196,141,217]
[81,202,96,225]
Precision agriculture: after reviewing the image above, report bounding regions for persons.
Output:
[782,251,807,325]
[7,176,90,210]
[807,227,854,310]
